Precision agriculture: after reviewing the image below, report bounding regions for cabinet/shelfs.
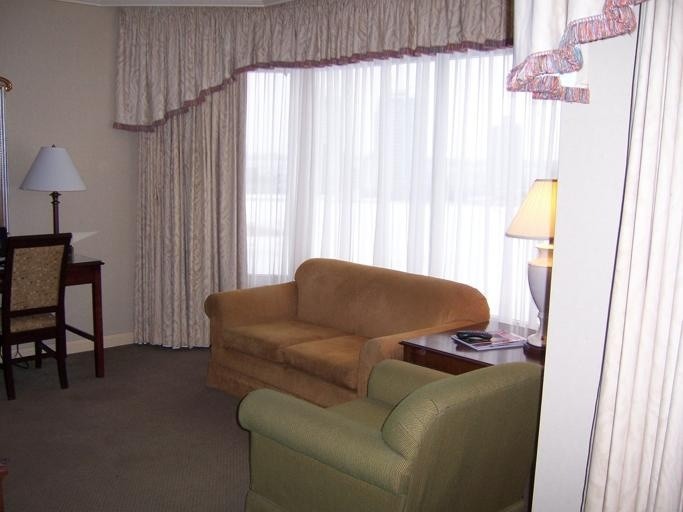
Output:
[400,320,546,376]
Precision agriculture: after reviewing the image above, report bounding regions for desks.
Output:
[0,256,105,376]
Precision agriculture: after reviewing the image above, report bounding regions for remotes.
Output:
[455,330,493,340]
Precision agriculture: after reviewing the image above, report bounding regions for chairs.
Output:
[237,360,544,512]
[0,232,73,400]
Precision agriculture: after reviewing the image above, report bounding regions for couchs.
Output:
[205,258,489,410]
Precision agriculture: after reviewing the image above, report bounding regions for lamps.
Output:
[504,179,559,360]
[18,146,86,234]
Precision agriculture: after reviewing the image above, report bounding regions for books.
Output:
[450,327,527,352]
